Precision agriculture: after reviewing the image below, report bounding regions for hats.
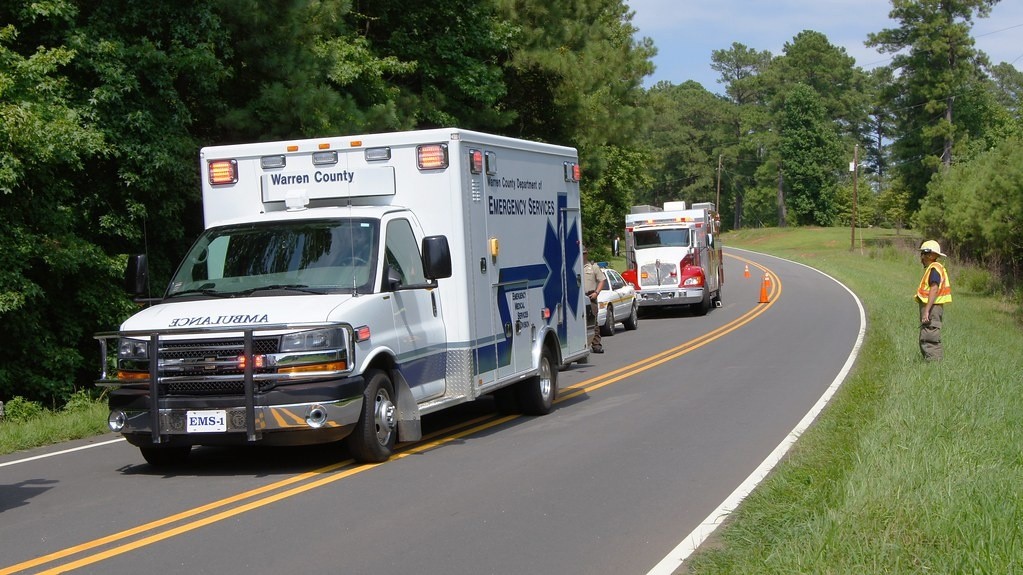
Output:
[582,245,587,253]
[917,240,946,256]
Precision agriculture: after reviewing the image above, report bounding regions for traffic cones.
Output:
[744,265,750,277]
[764,269,771,286]
[758,275,770,303]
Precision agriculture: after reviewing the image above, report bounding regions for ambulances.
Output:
[92,128,590,465]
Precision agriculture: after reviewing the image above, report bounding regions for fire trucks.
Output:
[611,201,725,317]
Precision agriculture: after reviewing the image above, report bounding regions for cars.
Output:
[593,261,638,336]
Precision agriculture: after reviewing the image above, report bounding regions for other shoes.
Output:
[593,349,604,353]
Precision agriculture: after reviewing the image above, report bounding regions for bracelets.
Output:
[596,290,600,293]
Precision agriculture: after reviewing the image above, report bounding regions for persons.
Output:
[582,245,603,353]
[916,240,952,362]
